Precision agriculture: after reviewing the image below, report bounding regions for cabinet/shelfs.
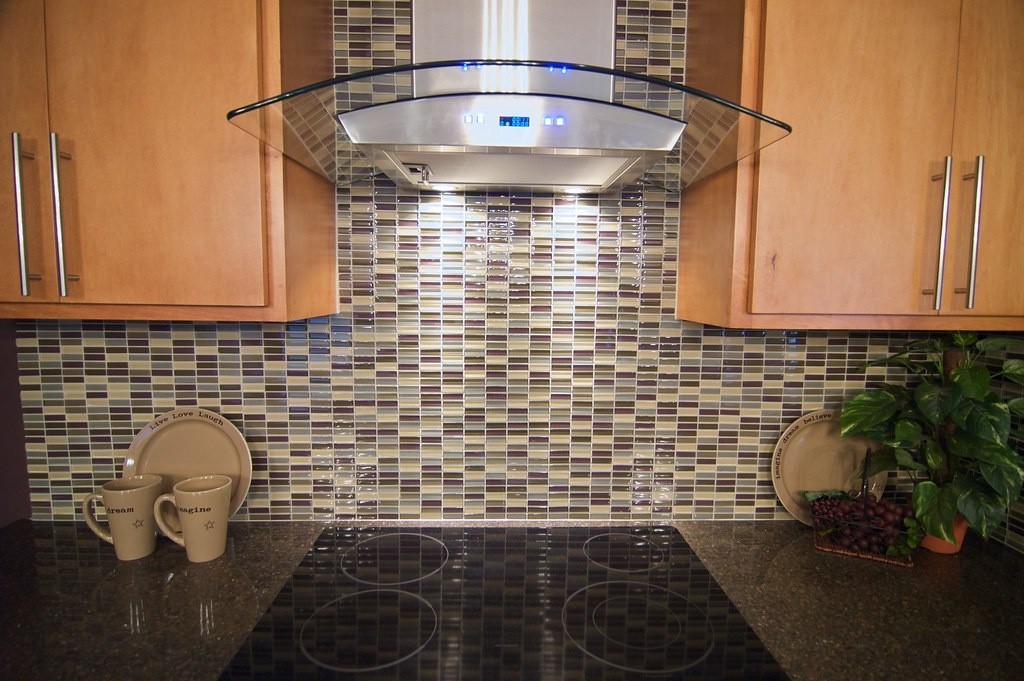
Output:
[677,0,1024,334]
[0,2,335,325]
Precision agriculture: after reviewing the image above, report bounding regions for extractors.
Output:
[224,1,795,198]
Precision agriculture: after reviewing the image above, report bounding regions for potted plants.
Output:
[837,330,1024,556]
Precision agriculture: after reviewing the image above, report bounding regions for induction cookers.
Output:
[217,526,793,681]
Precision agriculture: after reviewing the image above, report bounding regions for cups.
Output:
[153,474,232,563]
[82,474,162,561]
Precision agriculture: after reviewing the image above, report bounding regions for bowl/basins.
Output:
[806,501,927,568]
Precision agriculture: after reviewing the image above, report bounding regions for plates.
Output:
[774,407,890,527]
[122,406,255,537]
